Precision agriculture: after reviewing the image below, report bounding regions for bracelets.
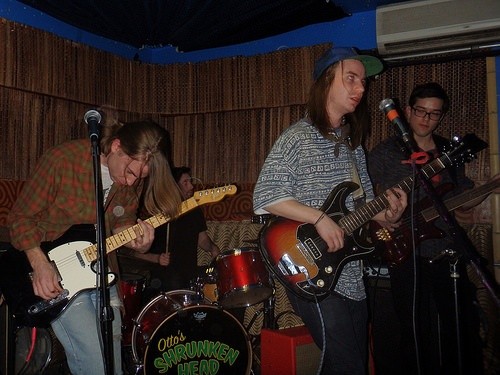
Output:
[313,212,326,227]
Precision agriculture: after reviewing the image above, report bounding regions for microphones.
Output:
[84,110,102,141]
[380,98,411,146]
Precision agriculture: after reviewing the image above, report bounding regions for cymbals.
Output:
[242,238,260,243]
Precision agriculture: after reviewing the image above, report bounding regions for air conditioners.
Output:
[375,0,500,65]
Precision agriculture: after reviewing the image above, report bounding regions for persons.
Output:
[9,115,176,375]
[367,79,499,375]
[133,164,220,296]
[252,42,409,374]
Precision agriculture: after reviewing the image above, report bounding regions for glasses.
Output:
[411,106,444,121]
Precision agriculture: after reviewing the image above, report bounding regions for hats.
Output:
[313,47,383,81]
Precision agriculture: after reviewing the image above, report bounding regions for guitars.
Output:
[0,183,237,326]
[257,134,488,304]
[361,176,500,266]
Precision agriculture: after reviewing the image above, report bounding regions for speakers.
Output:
[260,326,376,375]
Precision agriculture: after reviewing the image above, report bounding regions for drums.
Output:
[118,273,146,345]
[213,247,275,310]
[198,274,218,305]
[131,290,252,375]
